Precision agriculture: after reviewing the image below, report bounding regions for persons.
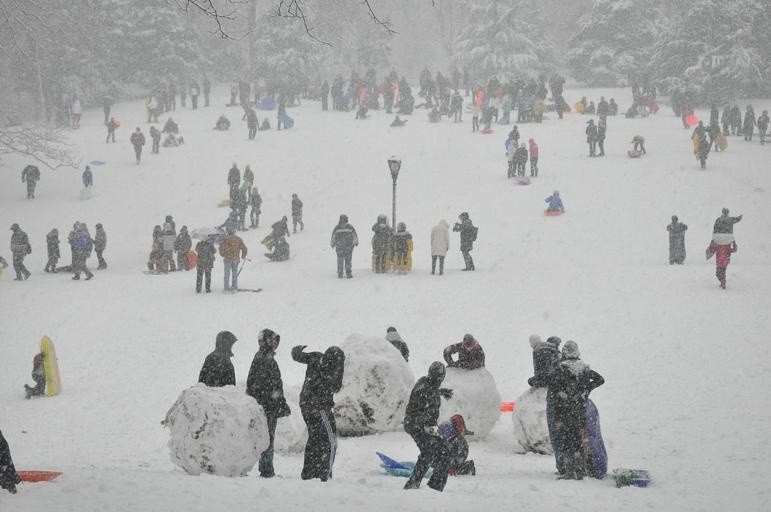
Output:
[0,61,771,297]
[289,344,348,482]
[438,412,478,478]
[246,328,292,479]
[0,429,23,496]
[525,332,560,386]
[442,332,488,370]
[22,350,46,398]
[541,340,607,480]
[404,360,455,493]
[547,334,562,349]
[383,324,411,364]
[198,329,239,388]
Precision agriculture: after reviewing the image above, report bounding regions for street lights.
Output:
[386,137,404,235]
